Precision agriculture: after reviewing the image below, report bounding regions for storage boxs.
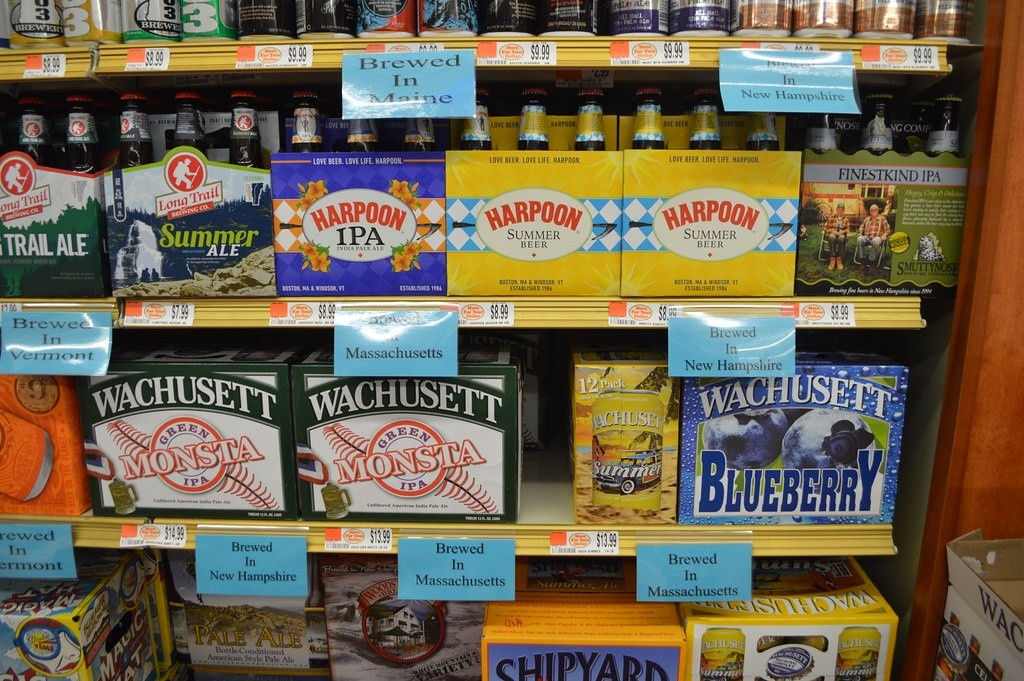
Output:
[75,338,313,521]
[794,144,972,294]
[0,120,121,297]
[291,342,523,524]
[565,332,680,526]
[459,328,552,450]
[677,329,910,525]
[0,547,900,681]
[934,529,1024,681]
[0,375,91,519]
[445,113,623,295]
[271,116,452,296]
[104,111,278,298]
[620,109,800,297]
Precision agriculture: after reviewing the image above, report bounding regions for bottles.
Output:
[18,88,963,156]
[932,612,1004,681]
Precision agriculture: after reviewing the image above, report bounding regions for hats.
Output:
[837,203,845,208]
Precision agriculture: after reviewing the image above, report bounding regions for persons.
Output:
[856,204,890,274]
[823,202,850,271]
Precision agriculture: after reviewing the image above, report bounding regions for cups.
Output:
[320,482,352,520]
[110,478,138,514]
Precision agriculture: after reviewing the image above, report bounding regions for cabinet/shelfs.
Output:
[0,36,950,555]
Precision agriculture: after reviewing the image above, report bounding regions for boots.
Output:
[836,256,843,270]
[828,256,836,270]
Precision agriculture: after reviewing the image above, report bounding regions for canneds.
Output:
[834,626,881,681]
[699,627,745,681]
[589,389,664,513]
[0,0,979,49]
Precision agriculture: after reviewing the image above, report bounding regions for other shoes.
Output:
[858,258,865,270]
[868,258,876,272]
